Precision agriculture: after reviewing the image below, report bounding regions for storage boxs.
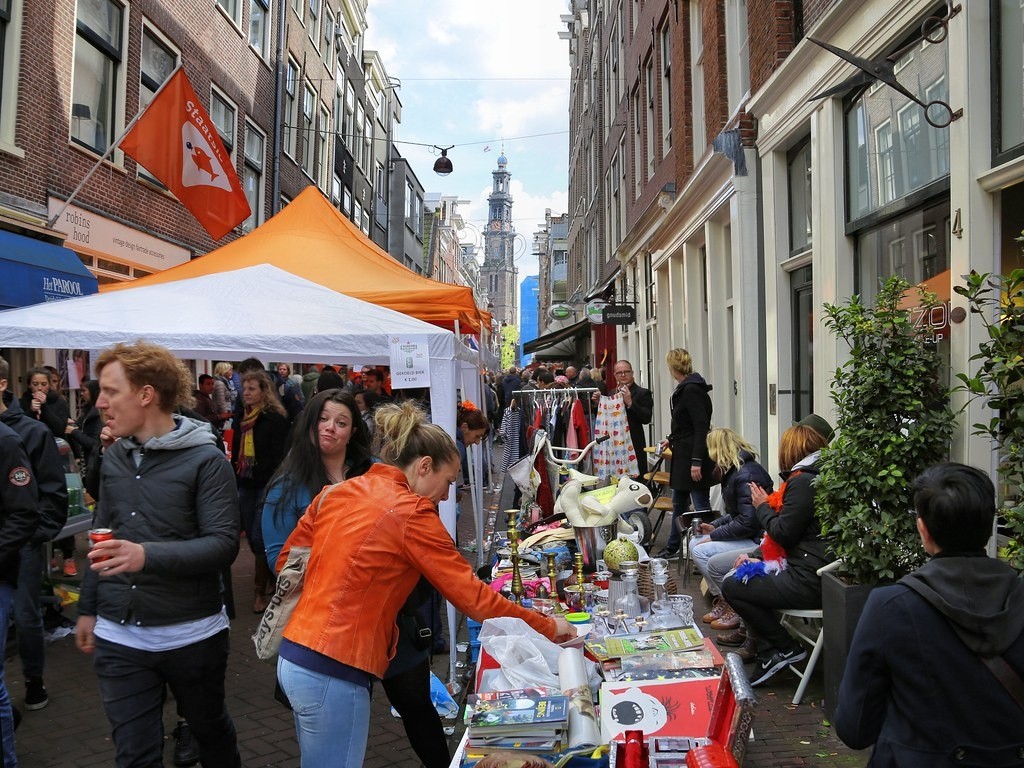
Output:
[609,653,757,768]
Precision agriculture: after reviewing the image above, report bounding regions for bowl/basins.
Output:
[564,583,608,612]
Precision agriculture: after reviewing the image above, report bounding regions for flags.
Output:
[118,68,252,243]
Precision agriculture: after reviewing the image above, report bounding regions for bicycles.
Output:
[535,428,611,520]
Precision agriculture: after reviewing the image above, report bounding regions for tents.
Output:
[0,186,497,695]
[876,268,951,342]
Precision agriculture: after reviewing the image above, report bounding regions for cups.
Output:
[667,595,695,628]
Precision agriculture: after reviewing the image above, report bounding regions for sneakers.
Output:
[653,548,680,562]
[702,597,741,629]
[457,481,471,489]
[23,678,50,710]
[749,640,808,687]
[170,719,200,768]
[716,630,746,647]
[734,631,762,665]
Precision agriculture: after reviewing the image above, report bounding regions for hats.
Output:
[792,414,836,448]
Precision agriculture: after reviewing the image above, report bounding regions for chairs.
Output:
[643,445,722,588]
[776,558,845,705]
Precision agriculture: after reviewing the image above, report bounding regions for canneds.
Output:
[87,528,114,572]
[66,488,81,516]
[692,518,702,536]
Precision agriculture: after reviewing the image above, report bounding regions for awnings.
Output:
[0,230,99,308]
[523,311,588,362]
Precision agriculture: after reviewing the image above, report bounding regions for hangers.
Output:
[509,386,578,410]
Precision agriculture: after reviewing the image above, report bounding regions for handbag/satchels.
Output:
[250,546,313,662]
[507,432,541,502]
[391,669,460,721]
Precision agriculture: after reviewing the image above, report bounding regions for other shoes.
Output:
[43,625,71,641]
[274,684,294,713]
[70,624,77,634]
[486,465,493,474]
[61,558,77,577]
[498,442,506,448]
[50,558,59,572]
[483,484,488,490]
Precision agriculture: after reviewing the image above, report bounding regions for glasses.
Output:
[615,370,632,377]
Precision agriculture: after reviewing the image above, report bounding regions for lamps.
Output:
[72,104,91,139]
[433,145,455,177]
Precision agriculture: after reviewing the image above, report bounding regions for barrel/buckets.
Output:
[572,519,620,572]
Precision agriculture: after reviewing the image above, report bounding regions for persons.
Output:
[0,357,70,710]
[691,425,774,630]
[833,463,1024,768]
[18,328,654,616]
[78,342,244,768]
[0,420,36,767]
[652,348,716,558]
[276,401,577,768]
[592,360,658,500]
[261,387,452,768]
[717,414,835,666]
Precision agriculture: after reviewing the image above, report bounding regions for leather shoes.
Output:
[267,582,277,594]
[252,588,267,614]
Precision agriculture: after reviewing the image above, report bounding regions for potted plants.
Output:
[810,275,966,731]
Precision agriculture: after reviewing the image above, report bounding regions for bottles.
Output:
[614,559,685,631]
[53,549,64,573]
